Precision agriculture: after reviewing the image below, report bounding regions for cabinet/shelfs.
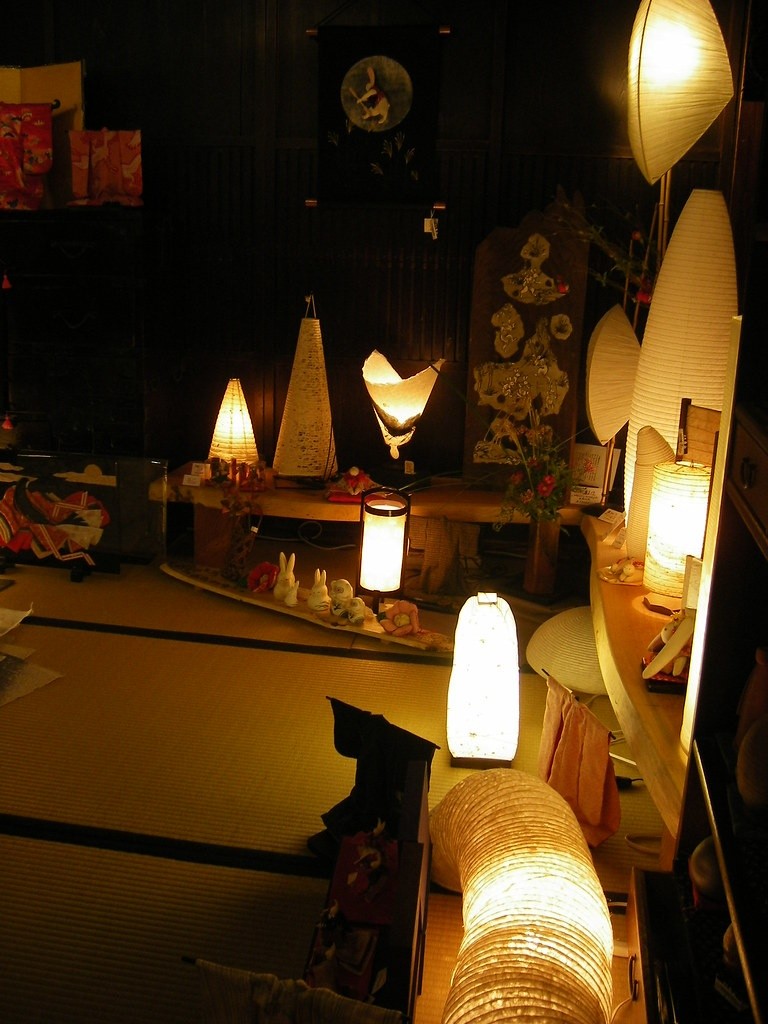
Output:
[624,0,768,1024]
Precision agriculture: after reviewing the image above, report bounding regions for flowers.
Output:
[497,398,574,538]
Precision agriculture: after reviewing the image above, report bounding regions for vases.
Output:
[522,514,565,593]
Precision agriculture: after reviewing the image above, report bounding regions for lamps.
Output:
[439,771,615,1024]
[525,605,612,698]
[353,487,411,616]
[272,295,344,491]
[205,379,261,484]
[444,592,522,771]
[360,348,447,493]
[587,0,741,621]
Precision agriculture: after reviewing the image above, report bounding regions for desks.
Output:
[143,457,582,606]
